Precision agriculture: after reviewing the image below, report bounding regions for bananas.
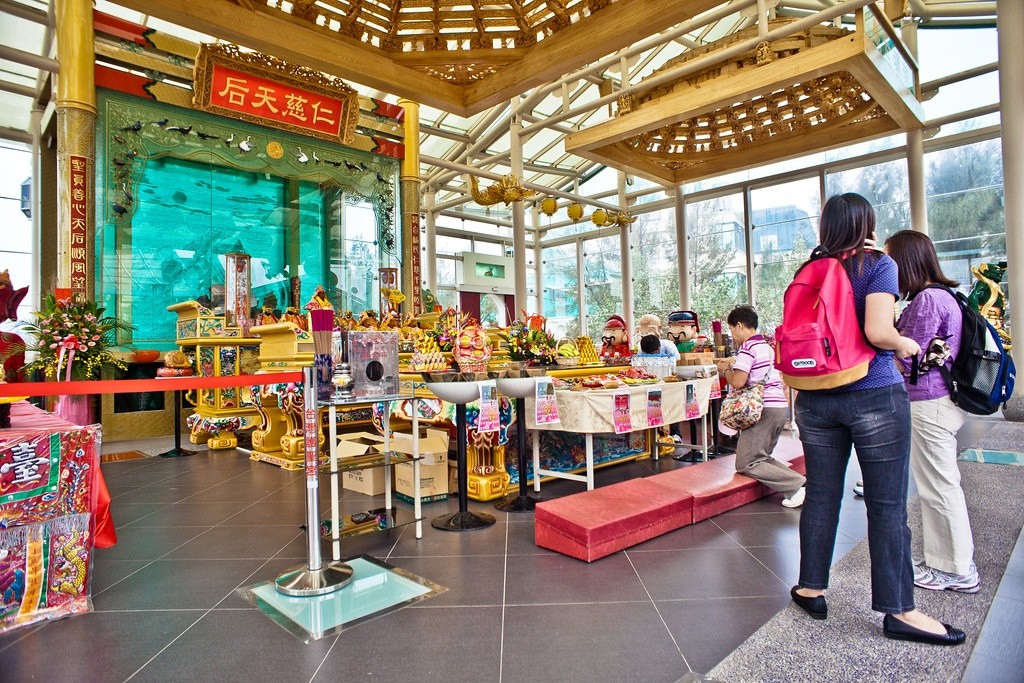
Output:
[558,343,580,358]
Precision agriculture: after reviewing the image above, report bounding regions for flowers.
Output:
[0,275,141,403]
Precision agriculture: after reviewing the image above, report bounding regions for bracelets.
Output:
[723,368,731,378]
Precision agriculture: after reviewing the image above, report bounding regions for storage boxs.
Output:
[335,429,460,506]
[678,352,716,365]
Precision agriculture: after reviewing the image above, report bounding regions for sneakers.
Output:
[911,557,982,593]
[781,487,806,508]
[671,434,683,443]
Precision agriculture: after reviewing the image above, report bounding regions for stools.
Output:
[427,375,550,532]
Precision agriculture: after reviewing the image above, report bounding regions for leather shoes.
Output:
[883,614,966,645]
[791,585,827,620]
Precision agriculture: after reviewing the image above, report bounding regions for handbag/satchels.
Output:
[719,380,766,431]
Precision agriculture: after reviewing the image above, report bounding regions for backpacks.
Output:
[773,244,882,395]
[916,283,1016,416]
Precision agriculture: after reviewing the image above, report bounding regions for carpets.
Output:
[698,419,1024,683]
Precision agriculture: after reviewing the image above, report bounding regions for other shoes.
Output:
[856,480,863,487]
[853,487,863,497]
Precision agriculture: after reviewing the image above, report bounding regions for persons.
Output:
[716,304,807,508]
[791,191,968,647]
[882,230,980,594]
[637,335,683,444]
[634,314,663,353]
[667,310,700,353]
[602,314,630,358]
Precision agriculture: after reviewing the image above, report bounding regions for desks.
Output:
[524,374,718,492]
[0,399,117,632]
